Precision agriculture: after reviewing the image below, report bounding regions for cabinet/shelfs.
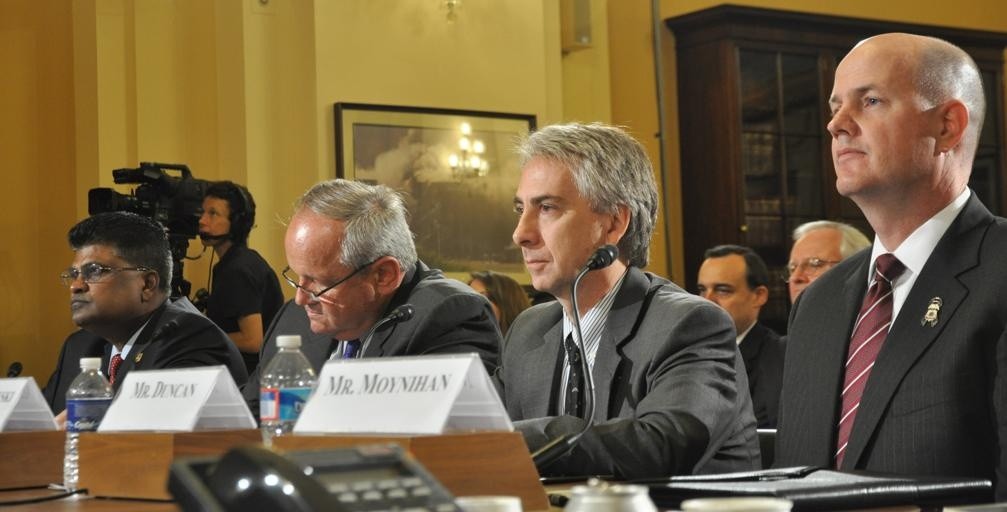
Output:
[664,4,1005,337]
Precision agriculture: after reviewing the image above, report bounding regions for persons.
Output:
[196,178,284,373]
[37,208,246,432]
[767,30,1007,499]
[469,269,533,333]
[692,240,787,467]
[239,174,505,432]
[781,220,876,308]
[489,123,768,480]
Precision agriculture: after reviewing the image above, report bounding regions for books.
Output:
[628,460,998,511]
[742,195,804,235]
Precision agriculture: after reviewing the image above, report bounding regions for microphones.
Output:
[7,362,22,378]
[532,242,618,472]
[358,303,415,359]
[201,233,229,242]
[113,319,179,392]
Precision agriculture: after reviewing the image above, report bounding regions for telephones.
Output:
[167,445,465,512]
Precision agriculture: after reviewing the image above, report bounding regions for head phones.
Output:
[211,180,255,243]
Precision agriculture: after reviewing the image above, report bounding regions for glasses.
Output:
[282,256,384,303]
[780,259,844,281]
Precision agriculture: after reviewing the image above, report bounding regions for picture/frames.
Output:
[332,101,543,297]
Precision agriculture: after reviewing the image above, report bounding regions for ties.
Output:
[562,331,585,417]
[108,354,123,384]
[833,250,908,469]
[339,336,362,362]
[62,263,160,282]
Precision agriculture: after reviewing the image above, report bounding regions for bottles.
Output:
[62,357,114,493]
[262,333,318,446]
